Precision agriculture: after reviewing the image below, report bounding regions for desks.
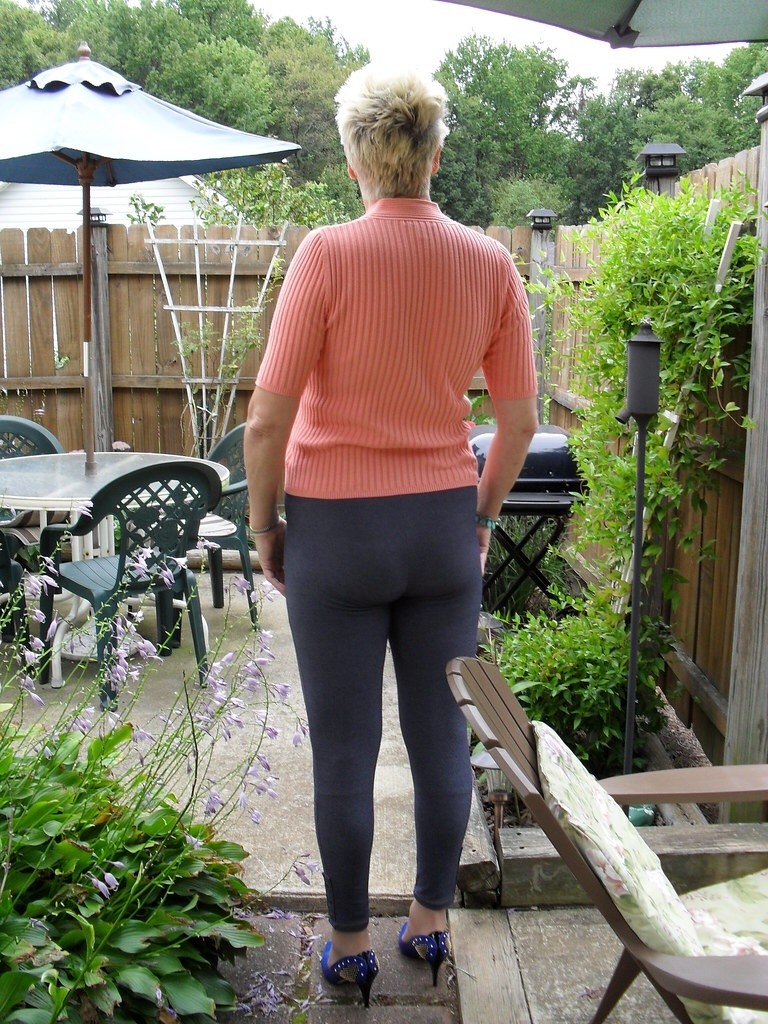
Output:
[3,451,231,664]
[474,502,582,618]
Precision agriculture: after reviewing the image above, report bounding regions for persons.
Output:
[243,60,543,1006]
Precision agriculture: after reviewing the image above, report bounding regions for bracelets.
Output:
[474,510,502,531]
[249,519,280,533]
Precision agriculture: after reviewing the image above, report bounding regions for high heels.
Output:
[400,922,453,987]
[323,941,380,1008]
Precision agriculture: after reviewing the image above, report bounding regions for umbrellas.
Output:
[0,41,302,478]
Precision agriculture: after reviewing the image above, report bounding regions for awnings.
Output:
[439,0,768,50]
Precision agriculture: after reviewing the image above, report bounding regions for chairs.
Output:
[204,423,261,632]
[36,462,223,714]
[1,417,70,574]
[447,657,768,1022]
[0,527,34,676]
